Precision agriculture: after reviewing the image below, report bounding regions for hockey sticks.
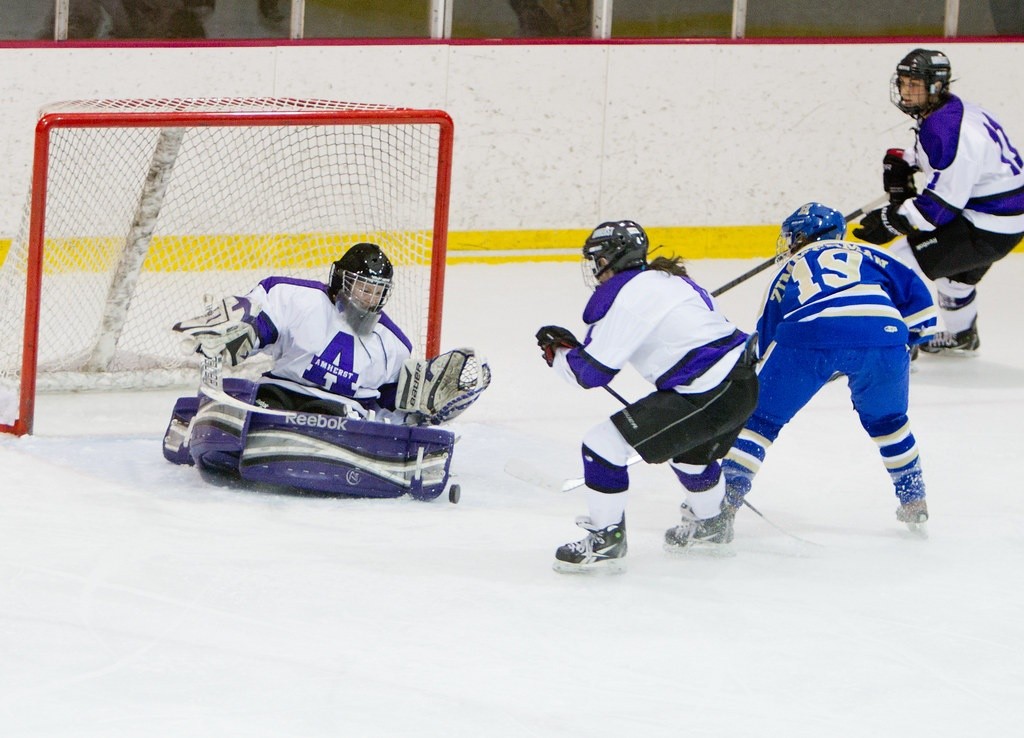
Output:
[534,325,768,518]
[709,189,891,297]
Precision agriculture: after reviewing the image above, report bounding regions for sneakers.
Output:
[918,314,981,360]
[664,497,744,560]
[550,510,628,577]
[893,470,929,540]
[904,325,922,373]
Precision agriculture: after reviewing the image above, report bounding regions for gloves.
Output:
[174,296,279,372]
[534,325,583,368]
[395,348,492,428]
[852,198,914,246]
[883,154,920,205]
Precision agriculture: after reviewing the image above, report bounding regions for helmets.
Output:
[584,222,649,285]
[327,244,393,336]
[782,204,848,259]
[891,50,951,121]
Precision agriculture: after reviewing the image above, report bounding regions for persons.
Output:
[165,241,492,494]
[682,201,934,546]
[24,0,601,47]
[854,59,1022,354]
[536,220,757,574]
